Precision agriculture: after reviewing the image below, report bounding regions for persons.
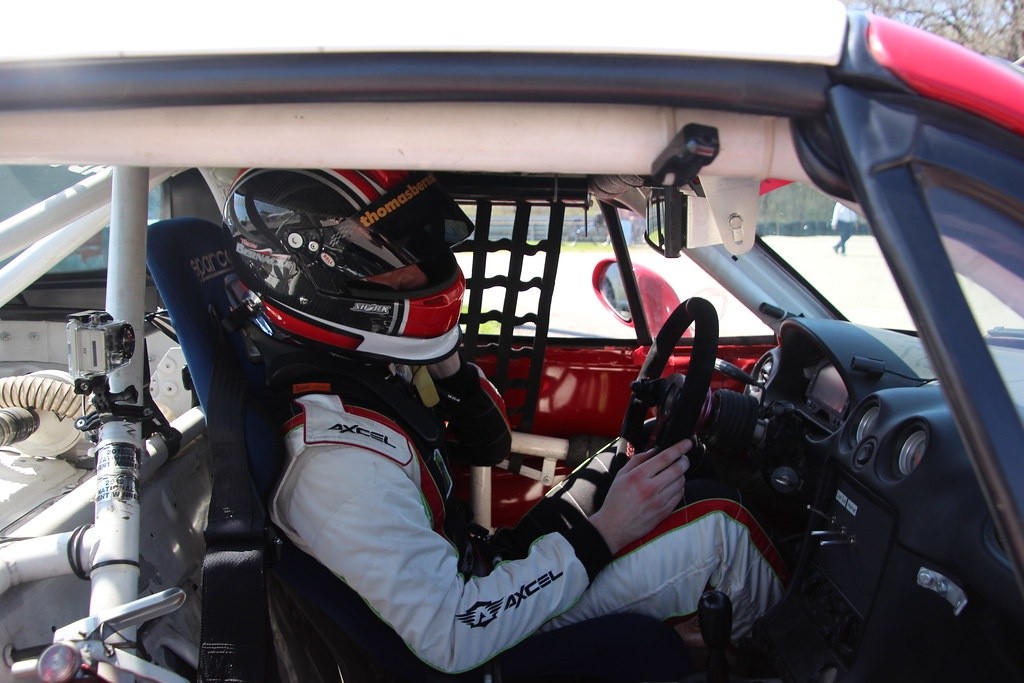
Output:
[603,208,645,246]
[831,201,859,255]
[222,168,801,683]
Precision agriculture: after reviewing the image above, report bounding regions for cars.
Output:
[0,0,1024,683]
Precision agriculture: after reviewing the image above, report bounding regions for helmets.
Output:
[221,166,474,366]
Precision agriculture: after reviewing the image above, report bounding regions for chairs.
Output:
[147,217,697,683]
